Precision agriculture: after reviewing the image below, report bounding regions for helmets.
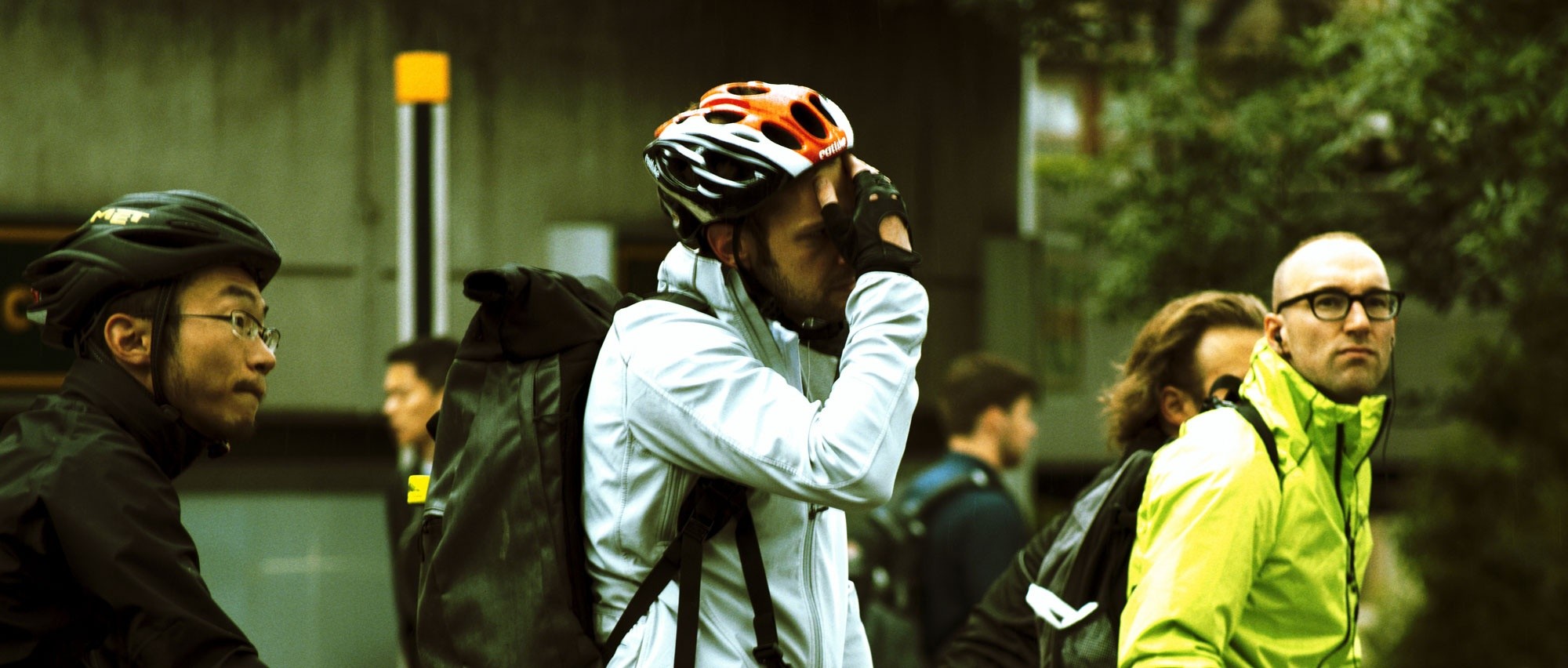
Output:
[645,76,857,246]
[27,189,281,343]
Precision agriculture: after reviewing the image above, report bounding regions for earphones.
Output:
[1273,331,1283,344]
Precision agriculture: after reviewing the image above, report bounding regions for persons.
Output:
[579,78,938,667]
[1115,230,1399,667]
[949,286,1273,668]
[379,336,463,668]
[0,190,286,667]
[868,355,1047,668]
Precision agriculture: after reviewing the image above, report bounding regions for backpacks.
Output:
[844,471,998,668]
[411,268,788,668]
[1024,453,1149,666]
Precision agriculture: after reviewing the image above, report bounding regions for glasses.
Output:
[185,309,282,352]
[1275,284,1402,324]
